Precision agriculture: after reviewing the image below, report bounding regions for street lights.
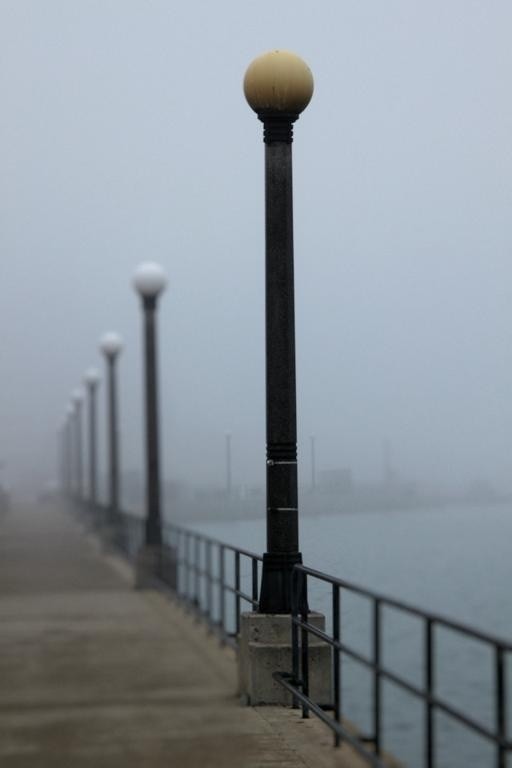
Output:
[236,51,336,708]
[56,368,103,533]
[130,259,178,590]
[99,331,127,554]
